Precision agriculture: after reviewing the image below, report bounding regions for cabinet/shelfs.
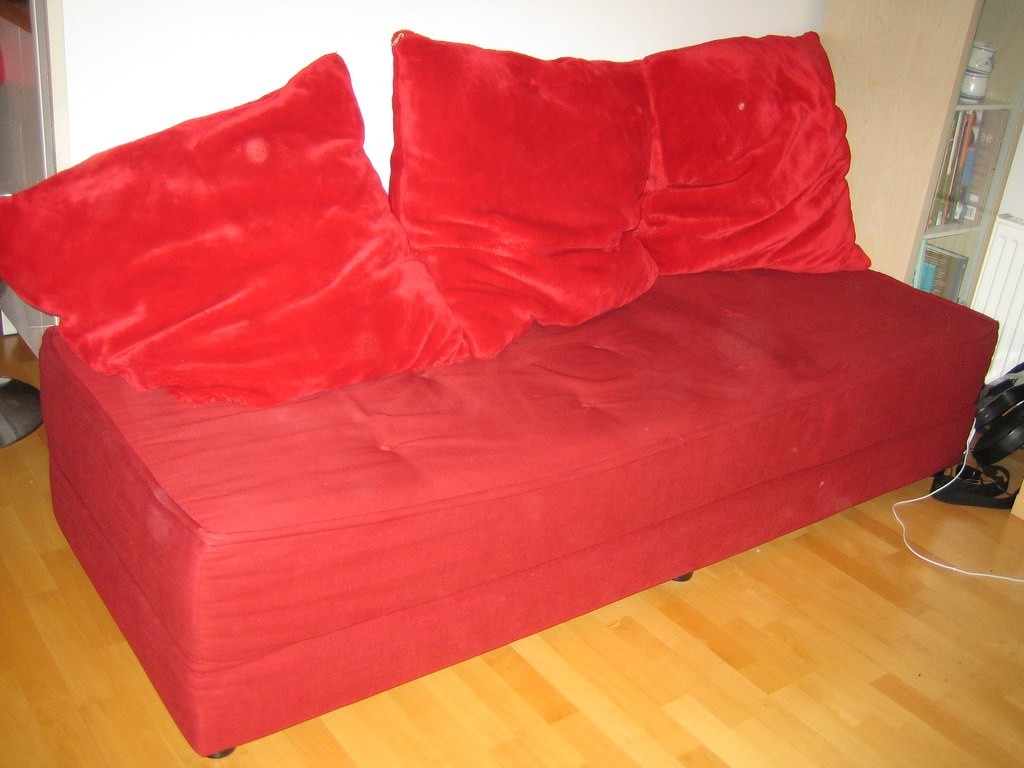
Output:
[0,16,56,358]
[819,0,1023,309]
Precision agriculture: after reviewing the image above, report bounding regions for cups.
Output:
[960,70,989,104]
[968,40,997,75]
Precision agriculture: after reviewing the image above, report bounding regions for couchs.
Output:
[41,271,999,759]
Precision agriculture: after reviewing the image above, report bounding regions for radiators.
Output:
[969,214,1023,385]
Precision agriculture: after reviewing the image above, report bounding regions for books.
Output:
[928,103,1010,227]
[921,244,969,302]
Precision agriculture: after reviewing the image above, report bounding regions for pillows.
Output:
[1,32,873,401]
